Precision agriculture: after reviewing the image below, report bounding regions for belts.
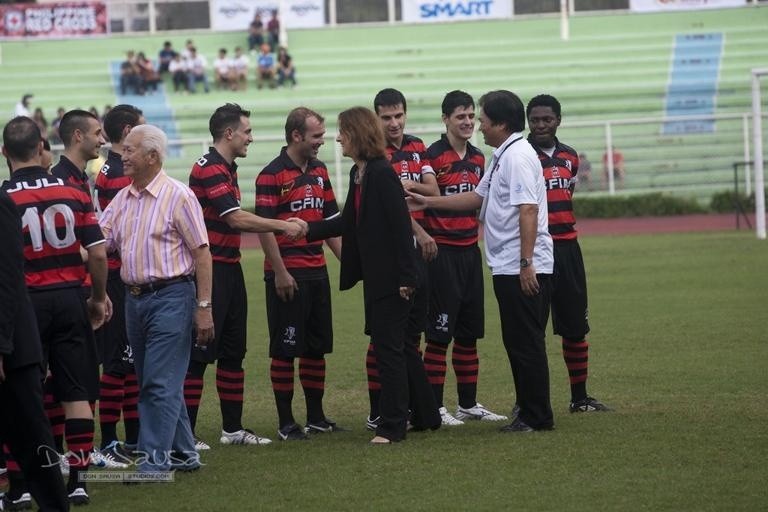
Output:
[120,275,197,296]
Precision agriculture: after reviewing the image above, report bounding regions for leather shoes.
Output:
[367,435,392,446]
[501,421,556,433]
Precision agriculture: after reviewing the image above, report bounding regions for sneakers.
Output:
[305,418,351,433]
[367,414,412,431]
[439,407,465,425]
[69,488,90,505]
[0,492,31,511]
[457,402,508,422]
[219,429,272,444]
[194,435,211,450]
[570,396,617,413]
[277,424,308,441]
[89,448,128,469]
[511,404,521,416]
[100,445,132,462]
[59,454,70,477]
[0,468,8,485]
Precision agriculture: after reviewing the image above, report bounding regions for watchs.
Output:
[520,258,532,267]
[195,299,212,308]
[0,116,106,512]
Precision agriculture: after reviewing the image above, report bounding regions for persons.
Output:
[366,89,441,433]
[283,107,418,444]
[268,10,280,46]
[512,95,616,417]
[93,104,146,464]
[256,105,354,441]
[402,90,555,432]
[181,40,193,58]
[186,48,209,93]
[15,94,33,118]
[229,48,248,91]
[51,108,68,144]
[103,105,112,123]
[184,103,301,450]
[247,15,264,49]
[600,146,620,187]
[93,123,216,483]
[49,110,129,475]
[158,41,176,72]
[213,49,234,90]
[120,50,140,95]
[31,108,47,141]
[423,91,508,425]
[575,154,594,192]
[1,186,70,512]
[274,47,297,84]
[168,52,188,97]
[256,43,278,89]
[88,107,102,123]
[137,52,158,96]
